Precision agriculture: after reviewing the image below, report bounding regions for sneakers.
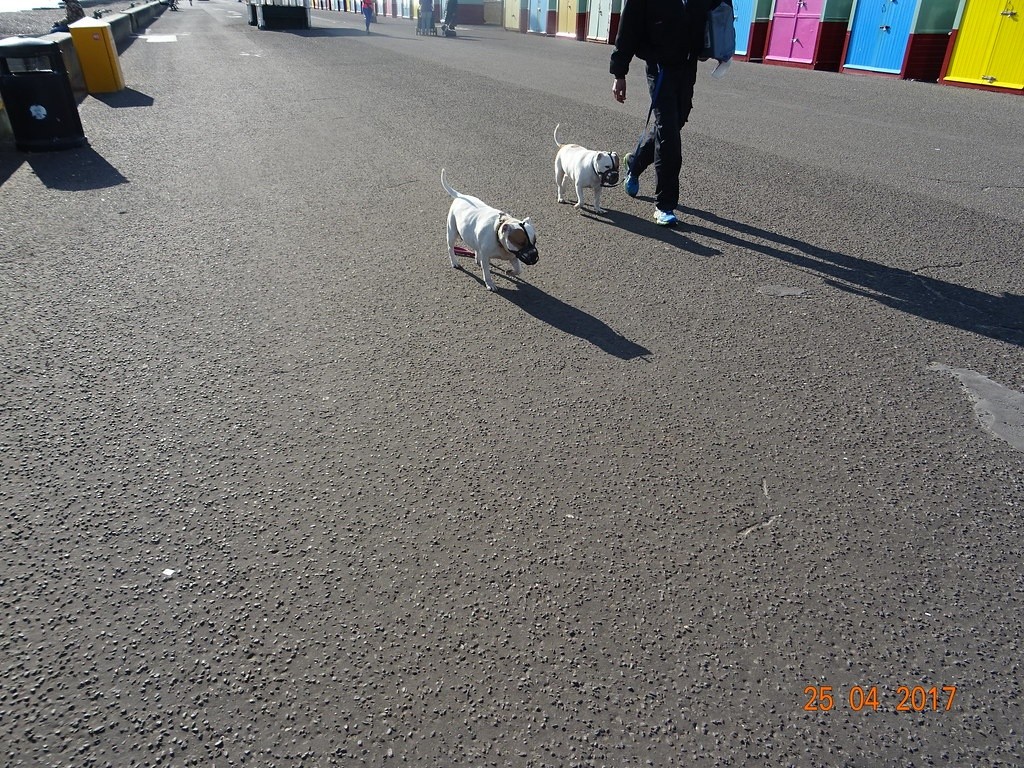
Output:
[653,206,676,225]
[624,152,639,197]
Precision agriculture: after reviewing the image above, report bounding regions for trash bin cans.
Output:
[0,34,86,151]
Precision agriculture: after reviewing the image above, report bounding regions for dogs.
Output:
[440,168,539,291]
[553,123,619,214]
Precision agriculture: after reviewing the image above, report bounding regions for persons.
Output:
[419,0,433,36]
[609,0,736,224]
[50,0,85,34]
[445,0,457,31]
[361,0,376,33]
